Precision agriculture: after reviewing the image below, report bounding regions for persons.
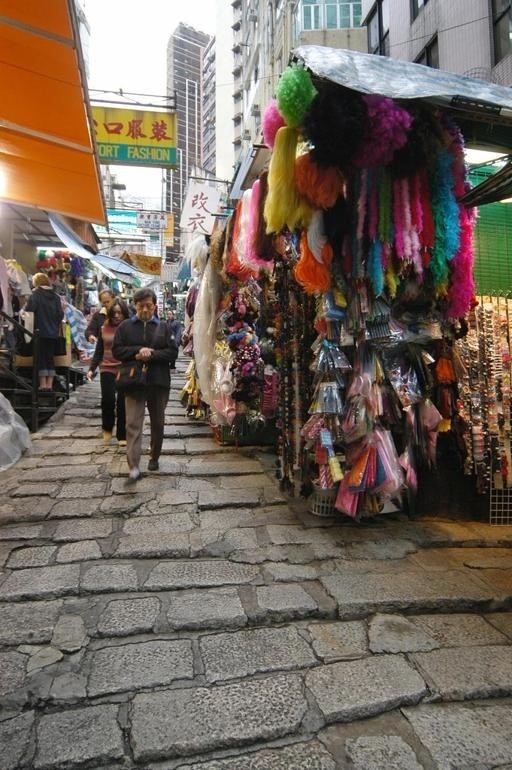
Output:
[10,288,24,325]
[111,286,179,479]
[126,300,183,369]
[85,297,130,446]
[25,272,66,391]
[84,289,114,345]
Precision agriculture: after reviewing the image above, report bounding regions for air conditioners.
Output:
[247,8,257,21]
[249,104,259,116]
[241,128,250,140]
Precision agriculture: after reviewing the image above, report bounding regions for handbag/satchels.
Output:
[114,359,153,392]
[55,336,67,357]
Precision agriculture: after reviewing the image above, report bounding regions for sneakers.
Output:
[129,458,160,480]
[103,431,127,447]
